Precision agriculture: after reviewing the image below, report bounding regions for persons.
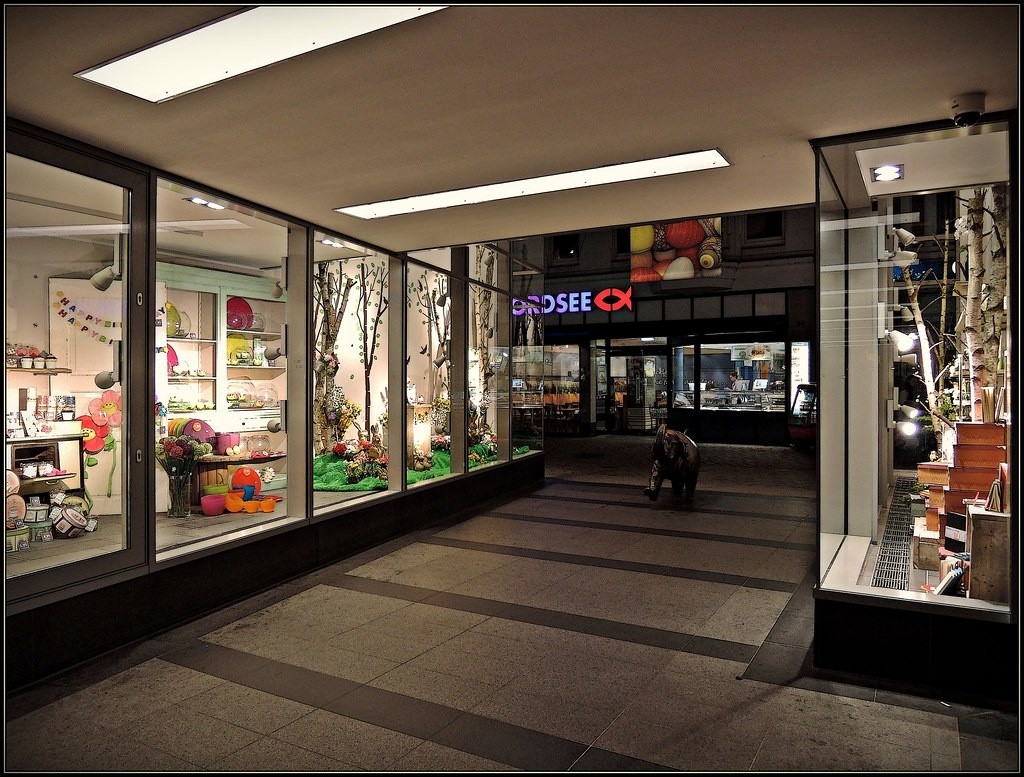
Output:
[729,372,747,404]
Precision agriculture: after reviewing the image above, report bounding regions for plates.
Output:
[168,419,191,437]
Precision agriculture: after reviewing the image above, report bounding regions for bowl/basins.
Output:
[201,484,275,515]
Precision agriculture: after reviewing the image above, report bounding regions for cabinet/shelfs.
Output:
[155,261,288,491]
[7,417,89,500]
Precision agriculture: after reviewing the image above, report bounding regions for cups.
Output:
[23,463,37,477]
[39,462,53,476]
[46,359,56,369]
[229,434,239,448]
[218,434,231,455]
[34,358,44,368]
[180,370,205,376]
[21,358,32,368]
[188,403,213,410]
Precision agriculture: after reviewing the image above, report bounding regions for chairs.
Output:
[512,400,580,433]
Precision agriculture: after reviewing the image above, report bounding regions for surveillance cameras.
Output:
[949,93,986,127]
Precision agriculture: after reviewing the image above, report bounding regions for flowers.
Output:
[155,435,213,518]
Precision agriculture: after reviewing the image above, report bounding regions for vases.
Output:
[167,475,191,518]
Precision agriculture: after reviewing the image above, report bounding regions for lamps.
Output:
[95,368,115,390]
[899,404,919,419]
[90,264,115,292]
[270,280,283,299]
[898,304,914,322]
[896,250,918,263]
[432,356,445,369]
[486,329,494,339]
[264,346,281,360]
[894,227,916,246]
[890,330,913,352]
[267,420,282,434]
[897,421,916,435]
[484,253,494,266]
[436,293,447,307]
[900,353,918,364]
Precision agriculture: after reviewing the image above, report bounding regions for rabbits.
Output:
[371,422,381,448]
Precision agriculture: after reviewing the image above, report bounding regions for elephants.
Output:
[640,424,701,506]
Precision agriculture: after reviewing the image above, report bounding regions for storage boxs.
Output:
[916,422,1008,552]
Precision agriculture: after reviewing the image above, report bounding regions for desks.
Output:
[512,405,543,432]
[187,453,251,506]
